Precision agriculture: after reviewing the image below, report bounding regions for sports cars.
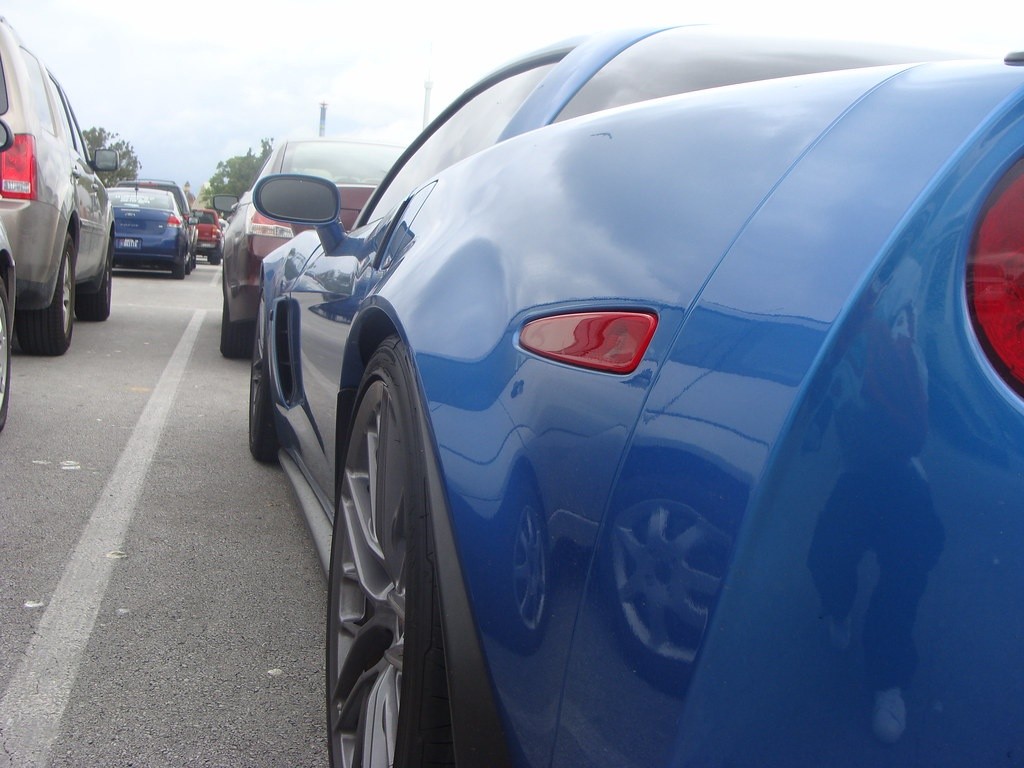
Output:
[248,19,1024,768]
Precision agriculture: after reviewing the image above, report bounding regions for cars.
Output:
[0,16,224,356]
[212,138,414,359]
